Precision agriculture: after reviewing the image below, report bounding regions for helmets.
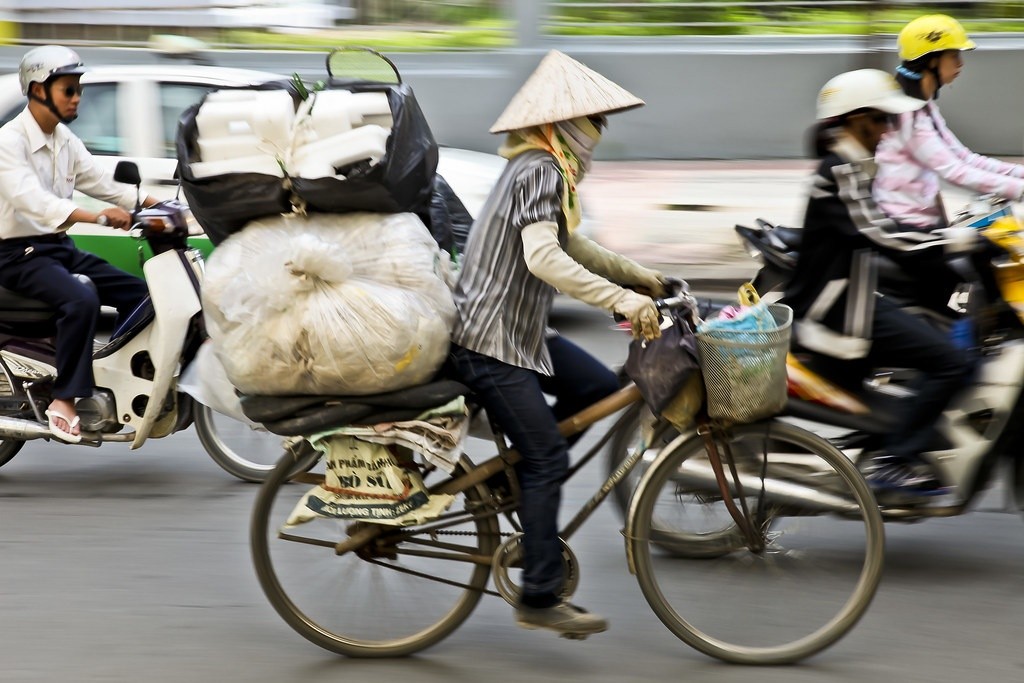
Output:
[898,14,978,61]
[19,44,86,95]
[815,69,930,119]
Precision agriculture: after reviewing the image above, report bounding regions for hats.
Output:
[488,48,646,136]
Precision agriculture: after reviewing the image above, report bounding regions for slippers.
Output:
[45,410,82,444]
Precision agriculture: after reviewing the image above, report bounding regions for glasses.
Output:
[42,84,83,98]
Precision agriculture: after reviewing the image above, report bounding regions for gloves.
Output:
[564,231,666,296]
[519,220,662,340]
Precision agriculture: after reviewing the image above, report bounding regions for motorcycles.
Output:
[601,195,1023,557]
[0,158,326,475]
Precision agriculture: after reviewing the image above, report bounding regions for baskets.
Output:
[695,301,794,421]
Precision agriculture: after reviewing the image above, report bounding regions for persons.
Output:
[792,14,1024,497]
[0,45,162,443]
[449,50,674,634]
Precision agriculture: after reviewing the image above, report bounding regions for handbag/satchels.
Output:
[624,308,705,433]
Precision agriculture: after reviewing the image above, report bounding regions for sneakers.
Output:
[853,445,940,493]
[517,603,608,635]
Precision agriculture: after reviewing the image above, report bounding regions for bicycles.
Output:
[249,287,887,667]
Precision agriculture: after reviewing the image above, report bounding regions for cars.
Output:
[1,35,597,278]
